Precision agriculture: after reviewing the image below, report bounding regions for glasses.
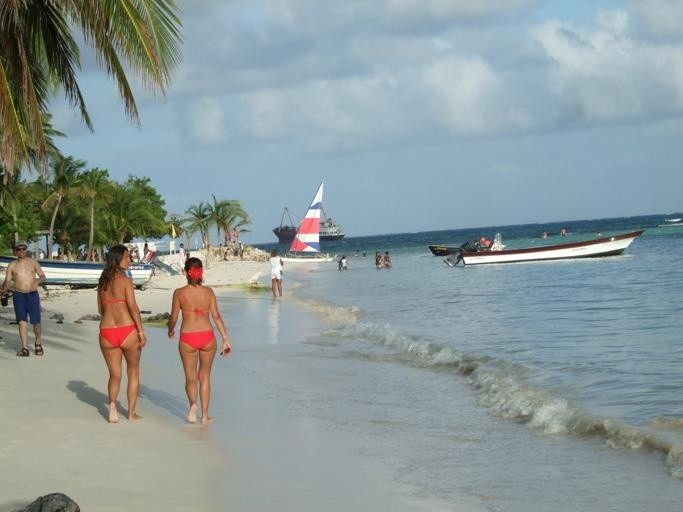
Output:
[16,247,26,251]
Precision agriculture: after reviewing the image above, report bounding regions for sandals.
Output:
[16,348,29,356]
[35,342,43,355]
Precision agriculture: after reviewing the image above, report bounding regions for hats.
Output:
[14,240,28,248]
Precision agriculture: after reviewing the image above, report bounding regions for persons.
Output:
[339,255,350,270]
[542,230,547,239]
[206,227,244,269]
[179,243,190,273]
[57,247,102,264]
[375,251,383,268]
[1,240,47,356]
[268,298,281,338]
[129,241,152,262]
[167,257,231,426]
[96,244,147,423]
[561,227,565,236]
[384,251,392,267]
[269,250,284,297]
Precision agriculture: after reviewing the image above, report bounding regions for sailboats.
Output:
[276,179,338,263]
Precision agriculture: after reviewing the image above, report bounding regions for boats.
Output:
[460,230,644,265]
[272,207,344,244]
[428,241,493,256]
[0,254,154,287]
[664,217,682,223]
